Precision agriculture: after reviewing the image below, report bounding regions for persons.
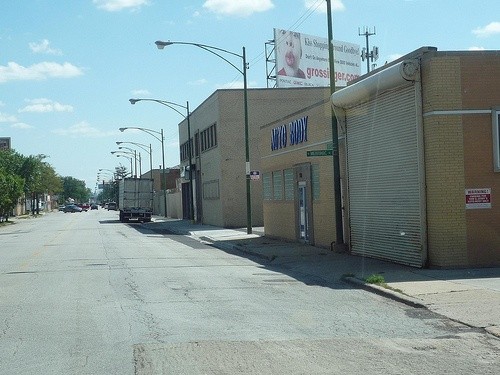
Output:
[278,29,305,78]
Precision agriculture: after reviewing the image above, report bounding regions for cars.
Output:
[58,202,98,213]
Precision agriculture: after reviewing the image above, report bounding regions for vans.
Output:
[104,202,118,211]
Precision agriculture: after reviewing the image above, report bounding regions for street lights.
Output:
[110,146,142,178]
[119,127,167,217]
[154,40,253,234]
[116,141,154,178]
[128,98,196,224]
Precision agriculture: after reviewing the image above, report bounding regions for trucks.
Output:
[118,178,154,222]
